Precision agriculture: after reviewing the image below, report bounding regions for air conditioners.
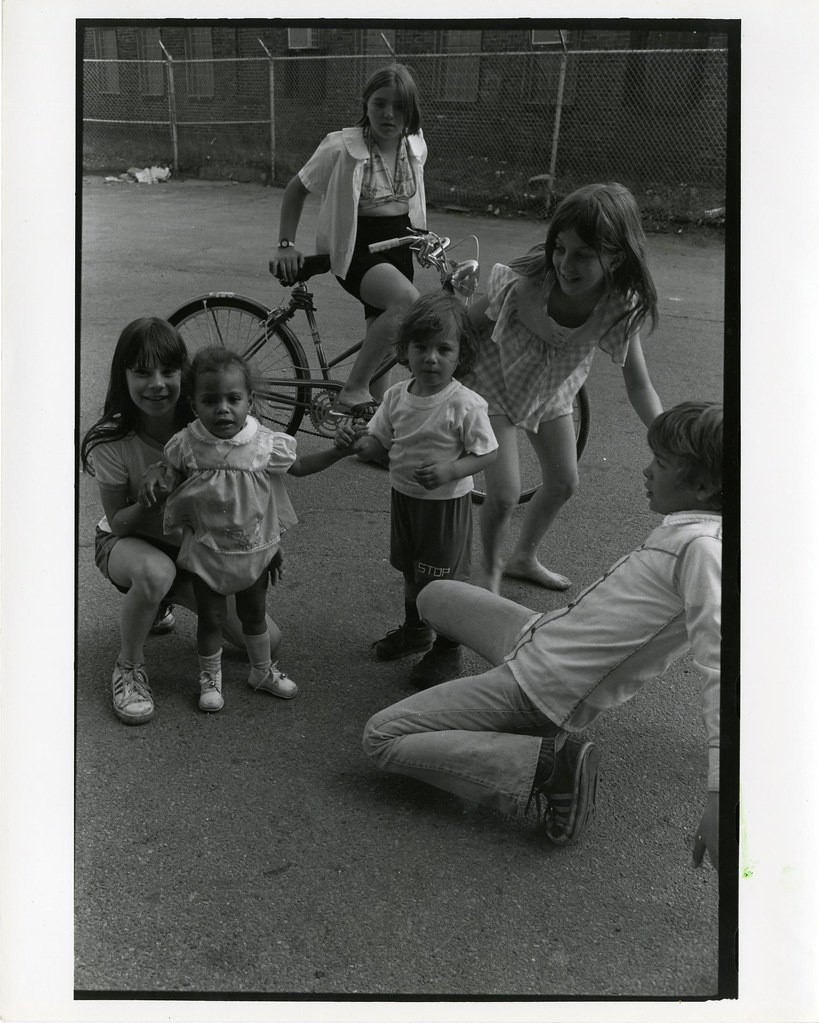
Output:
[532,30,572,46]
[288,26,323,50]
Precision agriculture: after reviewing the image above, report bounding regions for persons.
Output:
[82,316,282,724]
[138,342,370,712]
[272,63,428,420]
[334,291,499,689]
[361,400,723,878]
[466,182,664,596]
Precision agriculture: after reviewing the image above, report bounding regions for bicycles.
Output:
[169,224,591,506]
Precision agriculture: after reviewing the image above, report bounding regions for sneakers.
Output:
[111,655,156,725]
[408,640,464,691]
[152,603,176,632]
[373,619,434,659]
[249,660,298,699]
[196,668,224,711]
[532,732,599,847]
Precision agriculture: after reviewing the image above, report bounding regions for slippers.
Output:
[333,397,383,421]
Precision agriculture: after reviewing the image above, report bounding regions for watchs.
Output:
[277,238,294,248]
